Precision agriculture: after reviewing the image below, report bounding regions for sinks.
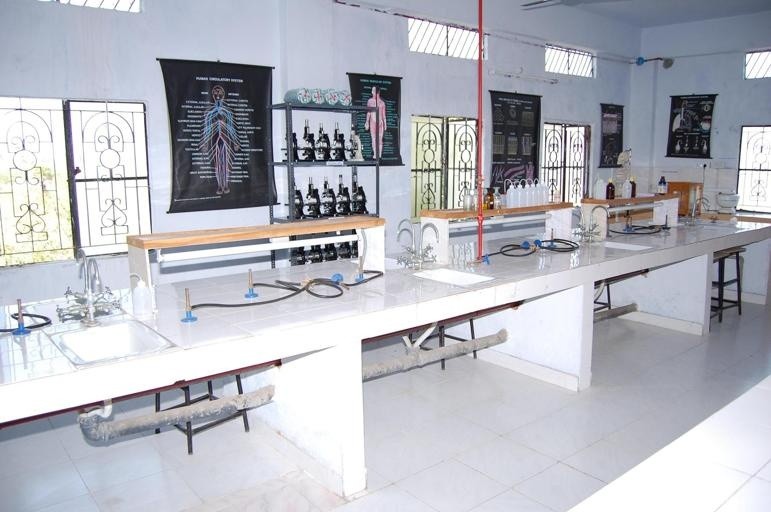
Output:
[411,265,496,289]
[703,223,740,230]
[348,253,408,273]
[593,239,655,253]
[54,314,174,367]
[26,289,121,330]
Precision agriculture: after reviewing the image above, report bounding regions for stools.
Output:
[710,246,746,324]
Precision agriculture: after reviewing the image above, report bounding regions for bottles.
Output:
[463,182,548,211]
[657,176,667,195]
[594,178,615,200]
[285,119,364,266]
[622,177,636,199]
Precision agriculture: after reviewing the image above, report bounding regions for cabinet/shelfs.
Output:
[264,101,380,268]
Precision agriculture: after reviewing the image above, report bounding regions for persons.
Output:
[364,85,388,160]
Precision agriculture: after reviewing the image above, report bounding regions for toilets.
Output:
[717,167,741,215]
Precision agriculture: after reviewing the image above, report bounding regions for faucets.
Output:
[691,196,711,227]
[395,216,419,272]
[78,257,104,296]
[417,221,441,271]
[589,204,612,244]
[73,247,102,326]
